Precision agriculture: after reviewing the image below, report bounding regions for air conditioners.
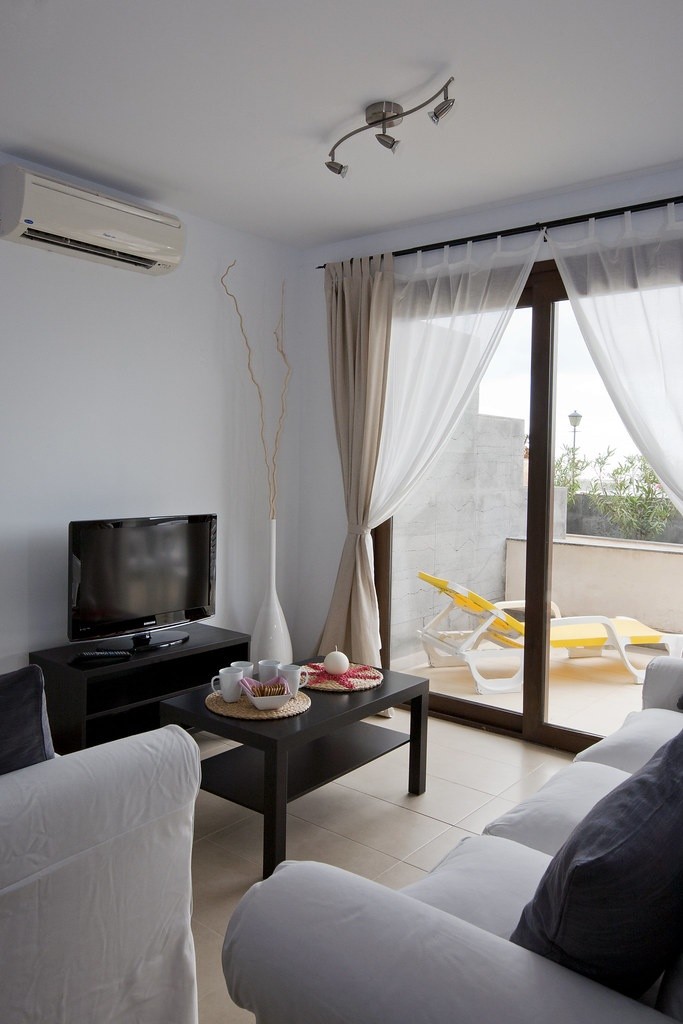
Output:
[0,160,185,278]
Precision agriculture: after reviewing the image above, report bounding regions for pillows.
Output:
[509,729,683,998]
[0,663,54,775]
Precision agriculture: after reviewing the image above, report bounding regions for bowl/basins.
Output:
[244,686,292,710]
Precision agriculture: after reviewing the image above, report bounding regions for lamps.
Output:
[325,76,456,178]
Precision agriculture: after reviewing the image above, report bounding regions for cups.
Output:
[278,665,309,699]
[258,659,280,685]
[210,667,243,703]
[231,661,254,694]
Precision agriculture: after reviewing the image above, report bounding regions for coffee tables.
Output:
[161,656,429,879]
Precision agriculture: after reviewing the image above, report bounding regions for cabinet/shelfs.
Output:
[27,622,251,754]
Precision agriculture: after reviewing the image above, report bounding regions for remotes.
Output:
[79,651,133,662]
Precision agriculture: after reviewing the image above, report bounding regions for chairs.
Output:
[414,572,683,694]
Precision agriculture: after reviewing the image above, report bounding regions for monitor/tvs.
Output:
[68,513,217,651]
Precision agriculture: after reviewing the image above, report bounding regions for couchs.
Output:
[0,723,201,1024]
[222,655,683,1024]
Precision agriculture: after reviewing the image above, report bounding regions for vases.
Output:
[249,520,293,673]
